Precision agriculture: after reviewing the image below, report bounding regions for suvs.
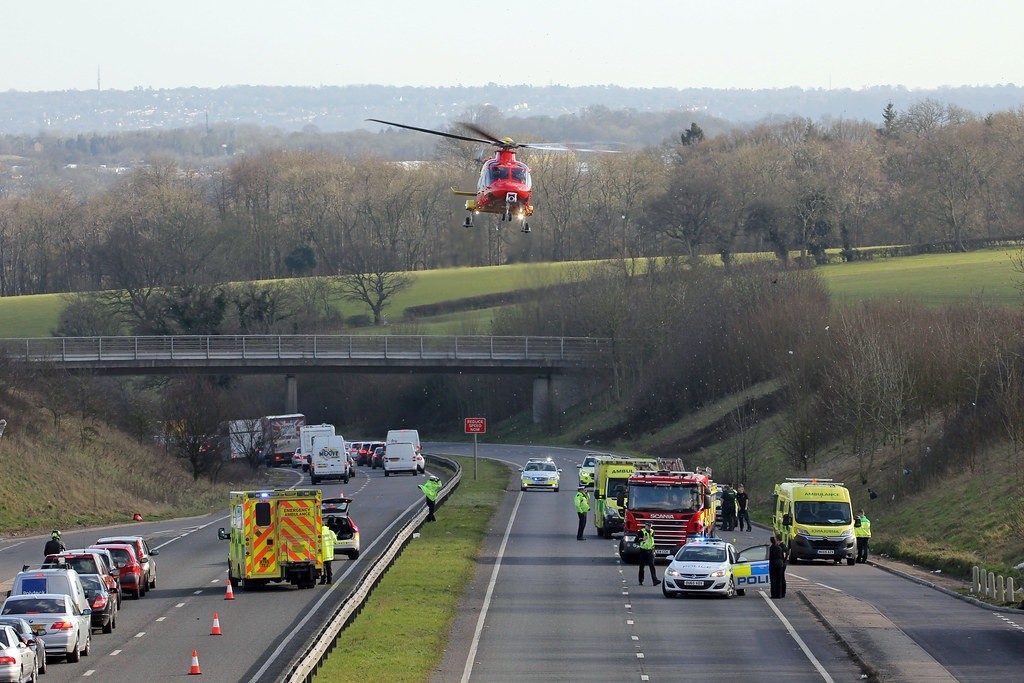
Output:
[7,563,92,640]
[41,536,159,614]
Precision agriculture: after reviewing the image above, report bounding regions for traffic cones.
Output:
[188,649,202,675]
[209,613,223,635]
[133,514,144,522]
[224,580,235,600]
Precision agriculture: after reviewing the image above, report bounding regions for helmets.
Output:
[51,530,62,540]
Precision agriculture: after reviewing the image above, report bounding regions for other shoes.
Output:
[653,580,661,586]
[577,537,586,540]
[326,582,332,585]
[770,595,785,599]
[718,527,734,531]
[639,581,644,585]
[318,581,326,585]
[427,518,436,522]
[746,529,751,532]
[740,527,743,531]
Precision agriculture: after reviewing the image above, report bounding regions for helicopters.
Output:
[365,118,629,233]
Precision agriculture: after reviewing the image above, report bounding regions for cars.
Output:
[322,498,360,560]
[0,594,92,662]
[416,451,425,474]
[155,435,217,453]
[576,453,613,487]
[0,616,47,683]
[662,538,770,598]
[345,441,383,469]
[78,574,119,634]
[291,447,301,468]
[716,484,738,526]
[518,457,562,492]
[346,452,358,477]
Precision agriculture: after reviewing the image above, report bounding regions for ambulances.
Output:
[217,490,323,591]
[773,477,861,565]
[595,458,659,538]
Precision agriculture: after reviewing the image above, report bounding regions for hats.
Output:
[645,520,654,526]
[577,486,585,491]
[430,476,436,479]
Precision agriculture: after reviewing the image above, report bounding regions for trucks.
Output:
[262,413,306,468]
[617,470,717,563]
[217,419,263,464]
[300,424,335,472]
[307,435,349,485]
[384,429,423,452]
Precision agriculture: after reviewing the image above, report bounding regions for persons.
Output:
[854,509,872,564]
[736,486,752,532]
[634,521,661,586]
[43,530,67,557]
[680,493,693,507]
[574,486,591,541]
[417,476,442,522]
[720,485,738,531]
[318,521,337,585]
[768,526,790,599]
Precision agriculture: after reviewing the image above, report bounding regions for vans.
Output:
[383,442,417,477]
